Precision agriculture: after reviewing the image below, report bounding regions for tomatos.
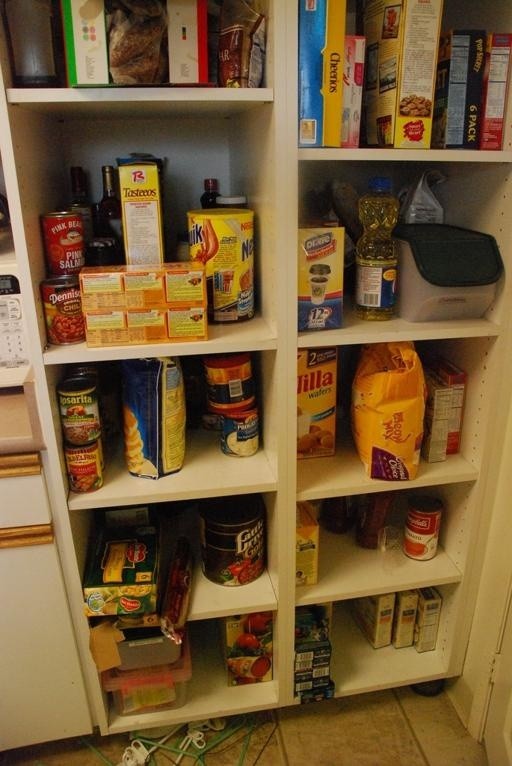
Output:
[236,613,272,685]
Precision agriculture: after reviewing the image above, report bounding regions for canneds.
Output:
[197,498,267,587]
[401,495,444,562]
[202,355,260,456]
[176,194,257,326]
[37,210,116,346]
[56,365,123,492]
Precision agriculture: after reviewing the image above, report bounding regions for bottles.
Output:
[68,165,96,243]
[355,176,399,322]
[95,162,122,238]
[199,178,222,209]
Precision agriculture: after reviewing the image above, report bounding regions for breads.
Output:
[105,0,168,84]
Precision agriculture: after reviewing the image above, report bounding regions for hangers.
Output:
[81,714,279,766]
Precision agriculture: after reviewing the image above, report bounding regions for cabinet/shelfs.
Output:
[0,0,511,765]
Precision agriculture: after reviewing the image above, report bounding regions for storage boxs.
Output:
[118,627,181,671]
[102,631,192,717]
[219,612,273,686]
[59,0,217,88]
[395,224,503,322]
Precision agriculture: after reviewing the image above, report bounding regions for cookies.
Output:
[399,94,432,118]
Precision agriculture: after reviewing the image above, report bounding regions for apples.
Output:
[125,543,149,563]
